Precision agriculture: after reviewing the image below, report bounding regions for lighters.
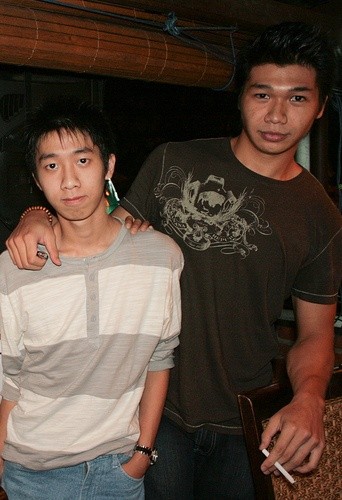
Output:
[37,249,49,258]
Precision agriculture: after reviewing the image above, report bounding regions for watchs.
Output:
[133,443,160,466]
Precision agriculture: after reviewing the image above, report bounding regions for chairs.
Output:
[236,363,342,500]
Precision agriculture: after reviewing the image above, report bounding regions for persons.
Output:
[5,20,342,500]
[0,93,186,500]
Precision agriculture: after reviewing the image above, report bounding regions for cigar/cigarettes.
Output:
[262,449,298,485]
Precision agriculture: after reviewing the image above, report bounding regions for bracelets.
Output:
[18,207,53,227]
